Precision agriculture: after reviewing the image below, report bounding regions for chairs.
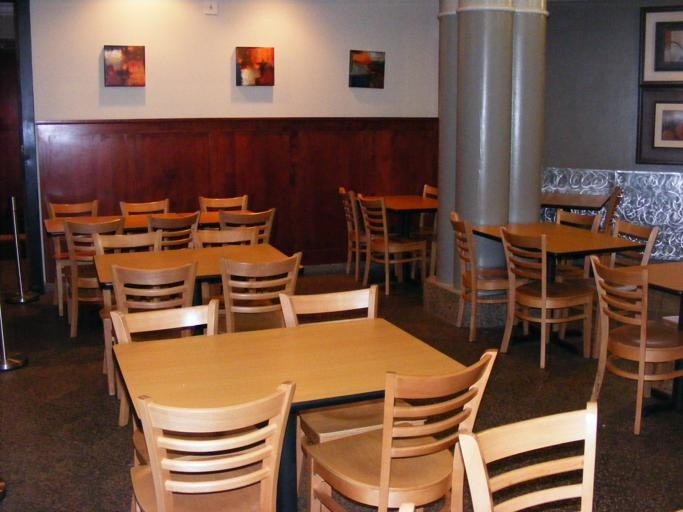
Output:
[459,400,598,512]
[300,347,500,512]
[126,382,295,512]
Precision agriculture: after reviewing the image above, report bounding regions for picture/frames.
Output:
[635,4,682,86]
[635,84,681,168]
